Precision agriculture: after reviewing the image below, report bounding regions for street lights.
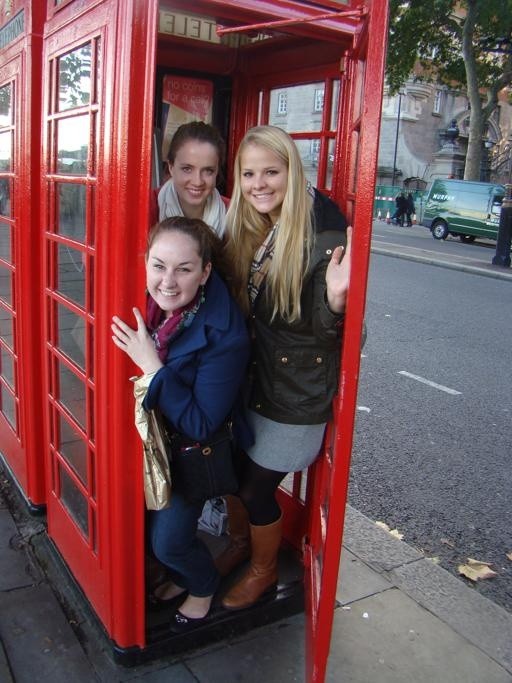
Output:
[391,87,408,196]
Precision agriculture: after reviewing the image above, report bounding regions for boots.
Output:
[212,494,250,577]
[221,505,284,610]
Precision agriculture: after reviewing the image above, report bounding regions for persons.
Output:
[388,192,407,227]
[109,214,254,631]
[405,191,416,226]
[144,119,231,246]
[216,122,355,611]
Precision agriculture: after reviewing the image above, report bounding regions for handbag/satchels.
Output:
[129,373,172,510]
[168,427,238,500]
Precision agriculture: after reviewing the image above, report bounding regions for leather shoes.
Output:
[169,583,220,633]
[145,581,188,606]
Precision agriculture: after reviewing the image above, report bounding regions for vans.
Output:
[423,177,506,242]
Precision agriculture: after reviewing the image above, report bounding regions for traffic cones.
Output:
[412,211,417,224]
[375,207,381,221]
[384,208,391,222]
